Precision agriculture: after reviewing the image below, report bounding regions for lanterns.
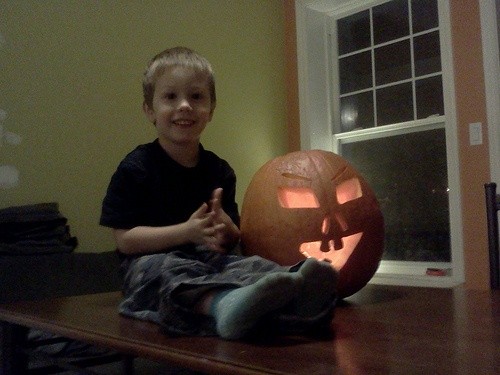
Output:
[241,149,385,299]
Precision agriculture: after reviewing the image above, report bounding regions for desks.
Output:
[0,252,500,374]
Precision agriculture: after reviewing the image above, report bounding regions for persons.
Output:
[98,46,339,341]
